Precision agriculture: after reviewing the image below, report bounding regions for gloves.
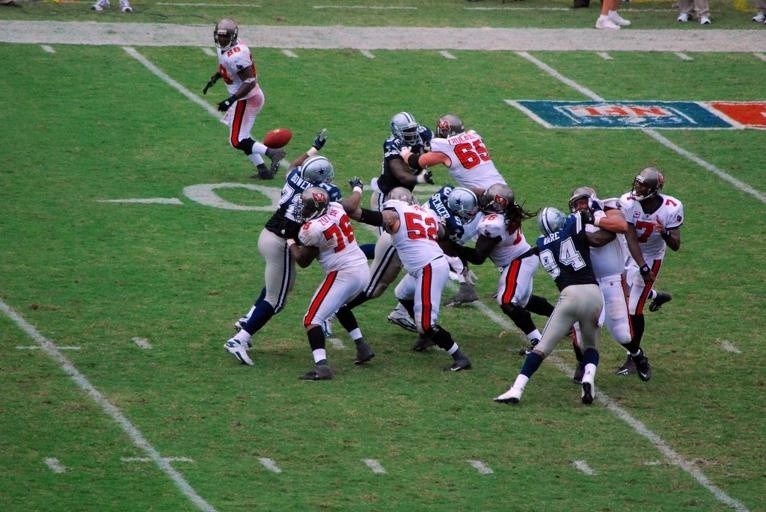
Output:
[203,80,213,94]
[217,95,238,112]
[347,176,364,190]
[312,129,328,151]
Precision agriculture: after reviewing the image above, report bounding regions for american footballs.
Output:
[263,129,292,147]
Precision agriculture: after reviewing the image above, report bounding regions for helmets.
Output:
[214,19,238,51]
[299,155,335,222]
[538,186,597,236]
[630,168,664,202]
[385,110,514,219]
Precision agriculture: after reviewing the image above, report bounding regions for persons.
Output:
[223,128,574,380]
[594,1,633,30]
[399,114,509,306]
[202,18,286,181]
[358,112,436,259]
[674,1,712,26]
[493,167,684,404]
[752,0,766,28]
[91,0,132,14]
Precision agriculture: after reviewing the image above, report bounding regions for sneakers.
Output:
[698,16,711,25]
[752,15,765,22]
[249,149,287,179]
[596,14,631,30]
[322,318,332,337]
[299,365,332,380]
[92,0,110,12]
[223,315,256,367]
[494,292,673,405]
[118,0,133,12]
[677,13,693,22]
[354,343,374,365]
[387,283,479,372]
